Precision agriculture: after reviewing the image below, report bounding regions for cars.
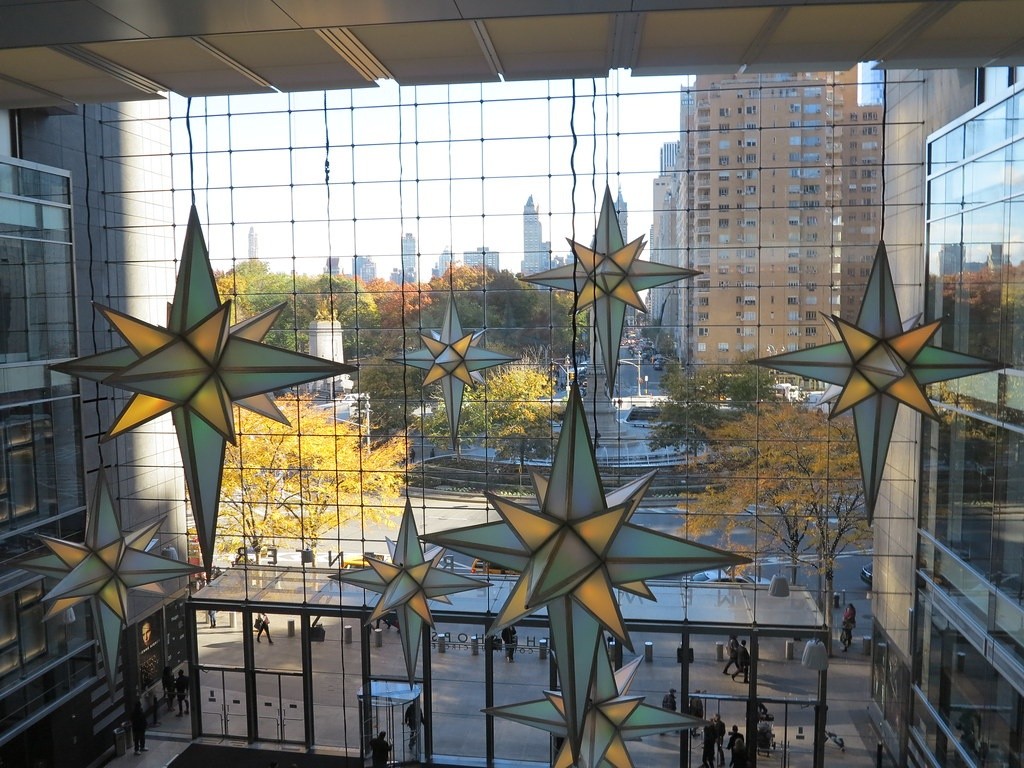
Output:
[684,569,770,584]
[860,561,872,586]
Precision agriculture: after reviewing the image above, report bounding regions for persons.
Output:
[197,565,222,590]
[731,639,750,684]
[840,603,856,653]
[501,624,516,662]
[256,612,275,644]
[689,688,746,768]
[756,700,768,724]
[122,689,149,756]
[175,670,189,718]
[161,666,177,712]
[208,610,217,628]
[430,448,435,457]
[722,634,739,675]
[405,698,425,750]
[409,448,416,464]
[659,688,680,736]
[368,615,400,634]
[369,731,393,768]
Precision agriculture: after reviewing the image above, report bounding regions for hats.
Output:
[669,688,676,693]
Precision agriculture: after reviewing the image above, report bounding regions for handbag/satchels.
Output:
[254,619,263,630]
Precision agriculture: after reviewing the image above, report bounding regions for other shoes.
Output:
[135,747,148,755]
[176,712,184,717]
[184,710,189,714]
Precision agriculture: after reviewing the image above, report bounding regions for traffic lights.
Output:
[267,548,277,565]
